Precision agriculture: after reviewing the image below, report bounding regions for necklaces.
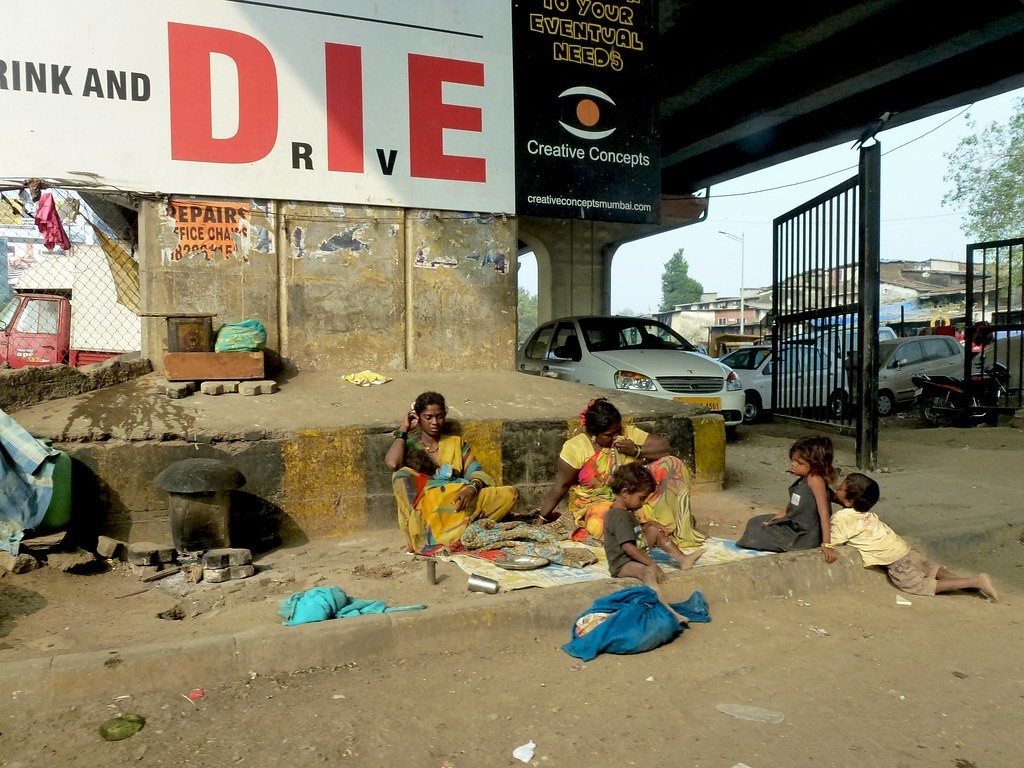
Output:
[419,436,439,453]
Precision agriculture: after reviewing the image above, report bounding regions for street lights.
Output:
[717,230,747,335]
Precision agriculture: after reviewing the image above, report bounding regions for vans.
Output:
[782,325,898,361]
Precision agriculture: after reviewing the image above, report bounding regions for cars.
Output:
[517,314,747,436]
[718,343,851,424]
[844,334,978,417]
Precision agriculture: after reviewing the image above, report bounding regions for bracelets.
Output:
[538,514,548,523]
[821,542,836,551]
[393,430,409,442]
[632,443,643,460]
[467,478,483,495]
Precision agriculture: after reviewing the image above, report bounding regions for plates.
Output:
[494,555,548,569]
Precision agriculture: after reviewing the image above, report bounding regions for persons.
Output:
[826,471,1001,604]
[602,462,707,630]
[384,391,519,555]
[735,434,840,564]
[533,396,642,551]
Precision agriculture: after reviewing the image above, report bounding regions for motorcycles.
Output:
[907,354,1019,429]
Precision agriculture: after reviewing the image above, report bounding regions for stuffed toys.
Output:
[630,414,706,551]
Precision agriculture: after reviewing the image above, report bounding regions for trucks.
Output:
[0,288,142,371]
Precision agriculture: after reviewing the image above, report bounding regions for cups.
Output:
[468,573,497,594]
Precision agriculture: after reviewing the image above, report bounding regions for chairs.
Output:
[564,335,593,351]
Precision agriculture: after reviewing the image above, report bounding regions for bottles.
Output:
[40,440,72,526]
